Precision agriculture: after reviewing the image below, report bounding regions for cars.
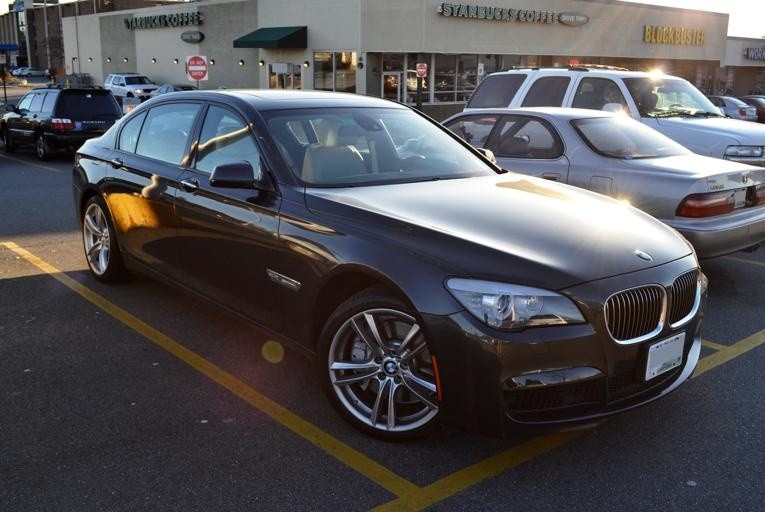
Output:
[397,99,765,268]
[20,67,45,77]
[736,93,765,119]
[706,92,759,122]
[148,82,199,99]
[64,86,723,448]
[383,68,476,101]
[11,66,28,76]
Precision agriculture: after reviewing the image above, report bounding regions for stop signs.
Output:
[416,63,427,77]
[185,55,209,81]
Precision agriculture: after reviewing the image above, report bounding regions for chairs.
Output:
[501,136,530,158]
[298,120,367,179]
[214,126,262,180]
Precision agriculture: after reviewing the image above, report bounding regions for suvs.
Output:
[0,81,126,161]
[105,70,160,101]
[462,63,765,166]
